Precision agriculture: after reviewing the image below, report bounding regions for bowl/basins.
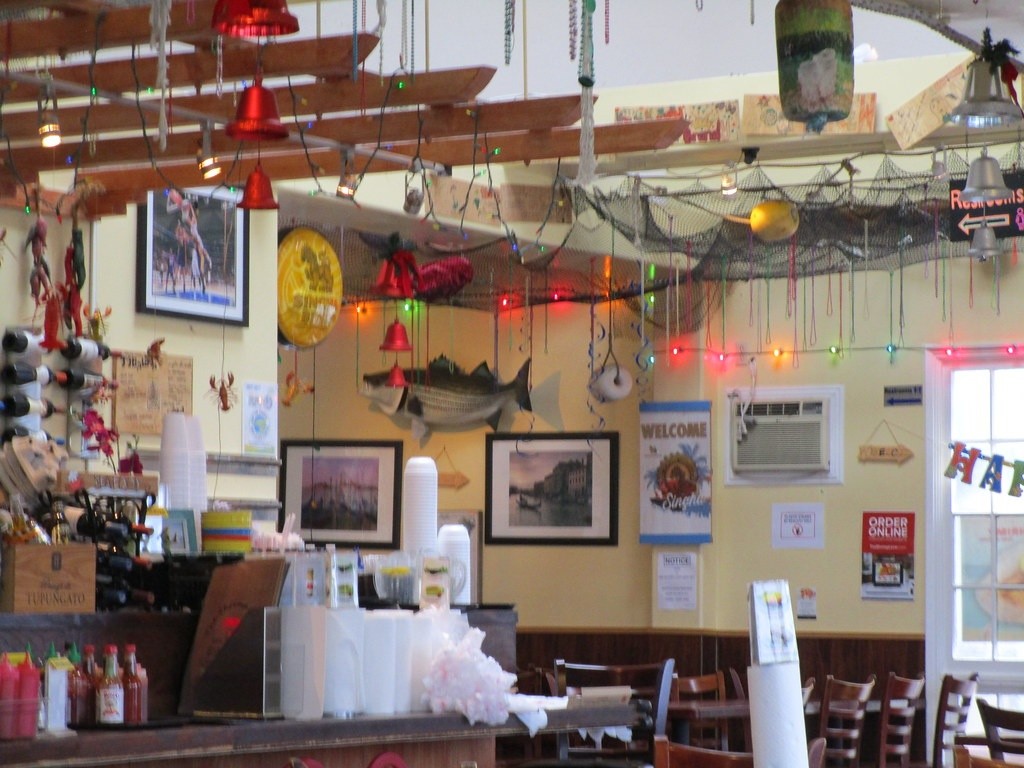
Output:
[399,457,472,605]
[161,413,207,511]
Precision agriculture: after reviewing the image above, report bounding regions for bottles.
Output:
[59,367,120,390]
[2,329,65,355]
[0,439,184,609]
[245,542,336,612]
[2,361,68,387]
[3,392,65,418]
[0,639,148,740]
[60,337,122,360]
[1,424,64,447]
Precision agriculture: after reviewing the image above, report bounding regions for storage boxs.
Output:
[0,541,96,618]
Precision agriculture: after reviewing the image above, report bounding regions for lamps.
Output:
[336,150,357,200]
[942,55,1024,130]
[959,146,1013,203]
[403,165,425,213]
[36,85,60,148]
[968,205,1001,257]
[195,117,221,178]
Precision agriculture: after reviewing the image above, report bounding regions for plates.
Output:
[202,509,253,551]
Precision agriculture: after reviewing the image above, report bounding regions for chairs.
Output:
[494,658,1024,768]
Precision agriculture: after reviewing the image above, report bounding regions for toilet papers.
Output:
[322,607,437,716]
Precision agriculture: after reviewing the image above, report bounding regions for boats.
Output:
[515,497,542,510]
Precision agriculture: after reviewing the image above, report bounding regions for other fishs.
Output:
[358,353,533,443]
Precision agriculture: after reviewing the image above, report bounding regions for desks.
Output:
[676,696,909,768]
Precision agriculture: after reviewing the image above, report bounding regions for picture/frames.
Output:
[135,183,250,324]
[485,430,620,546]
[278,438,403,549]
